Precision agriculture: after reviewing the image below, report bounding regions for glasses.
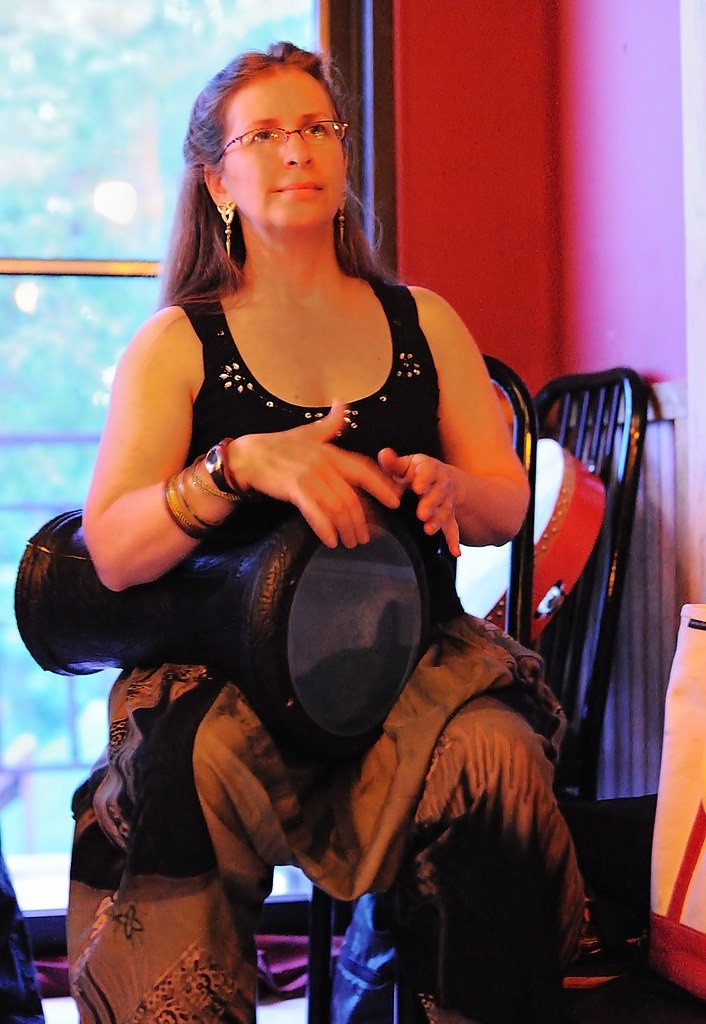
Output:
[216,120,350,163]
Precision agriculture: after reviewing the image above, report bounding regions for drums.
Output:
[449,438,605,645]
[12,507,428,757]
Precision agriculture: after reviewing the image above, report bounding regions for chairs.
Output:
[534,363,650,806]
[295,352,536,1022]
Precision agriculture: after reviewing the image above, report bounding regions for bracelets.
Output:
[180,466,226,528]
[163,471,212,542]
[192,453,243,503]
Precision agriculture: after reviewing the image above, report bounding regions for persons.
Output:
[64,40,593,1024]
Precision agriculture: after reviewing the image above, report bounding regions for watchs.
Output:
[205,437,248,500]
